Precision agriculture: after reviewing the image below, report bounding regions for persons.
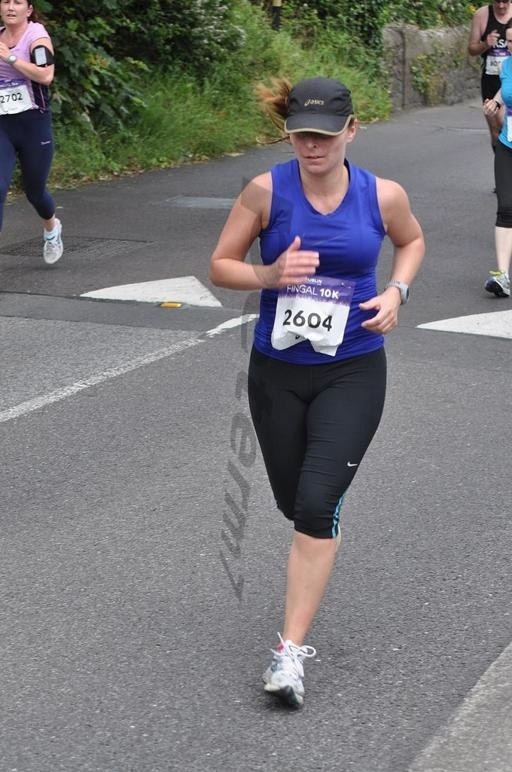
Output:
[1,1,67,265]
[207,75,428,709]
[478,17,511,299]
[468,1,512,194]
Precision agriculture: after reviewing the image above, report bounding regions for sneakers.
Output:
[41,220,63,264]
[260,638,305,707]
[484,271,509,297]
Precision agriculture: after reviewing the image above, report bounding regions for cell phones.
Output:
[34,47,48,66]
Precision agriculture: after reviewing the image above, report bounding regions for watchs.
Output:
[384,279,410,305]
[6,54,19,67]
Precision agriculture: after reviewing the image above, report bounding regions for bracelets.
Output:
[494,99,501,110]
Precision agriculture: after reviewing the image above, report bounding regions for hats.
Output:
[285,76,353,137]
